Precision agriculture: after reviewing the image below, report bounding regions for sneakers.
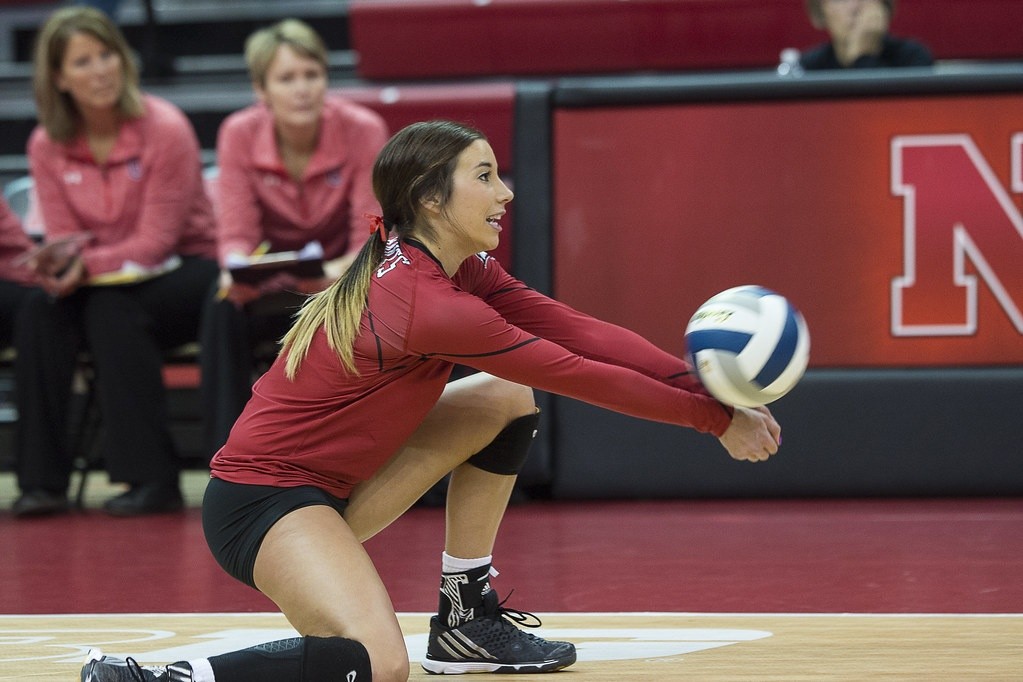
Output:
[80,648,193,682]
[420,616,577,673]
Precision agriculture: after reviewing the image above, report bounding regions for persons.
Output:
[786,0,936,71]
[78,117,785,682]
[9,4,218,518]
[195,16,393,468]
[0,192,36,352]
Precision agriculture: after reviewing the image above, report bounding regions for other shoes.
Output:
[14,493,57,514]
[105,483,186,515]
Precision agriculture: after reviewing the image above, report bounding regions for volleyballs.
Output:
[684,284,810,408]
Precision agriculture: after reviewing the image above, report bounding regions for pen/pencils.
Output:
[213,242,271,304]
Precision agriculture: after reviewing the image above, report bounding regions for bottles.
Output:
[777,48,803,77]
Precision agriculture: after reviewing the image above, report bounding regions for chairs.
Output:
[5,162,219,509]
